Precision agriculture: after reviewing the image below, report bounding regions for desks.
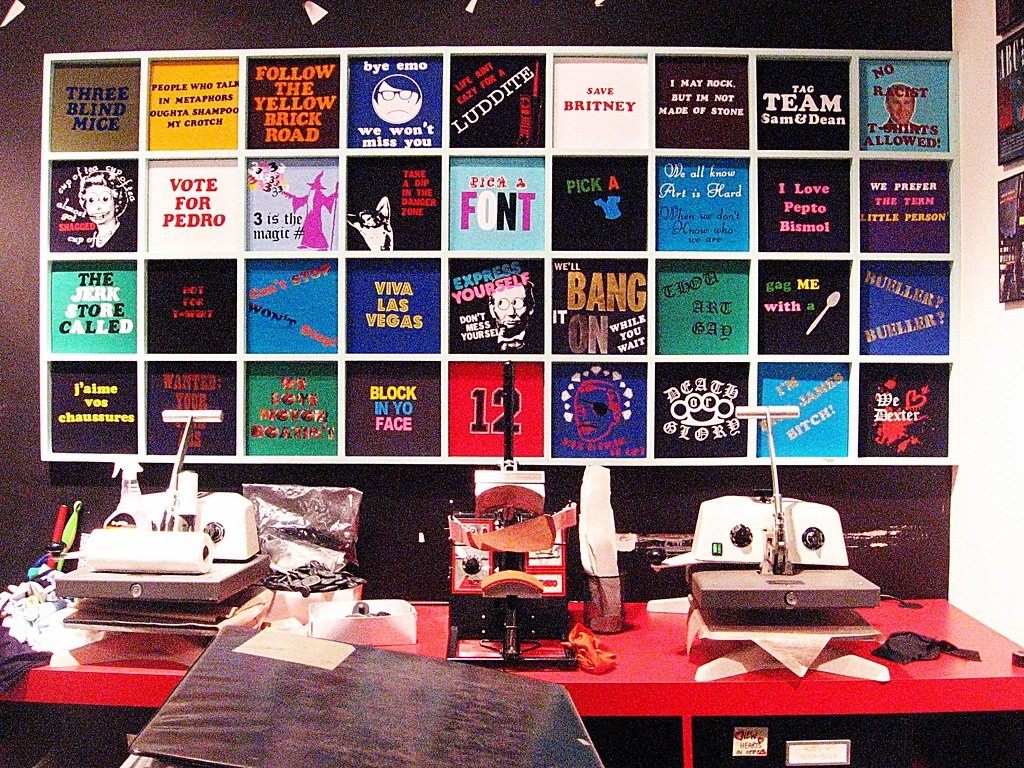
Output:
[0,599,1024,767]
